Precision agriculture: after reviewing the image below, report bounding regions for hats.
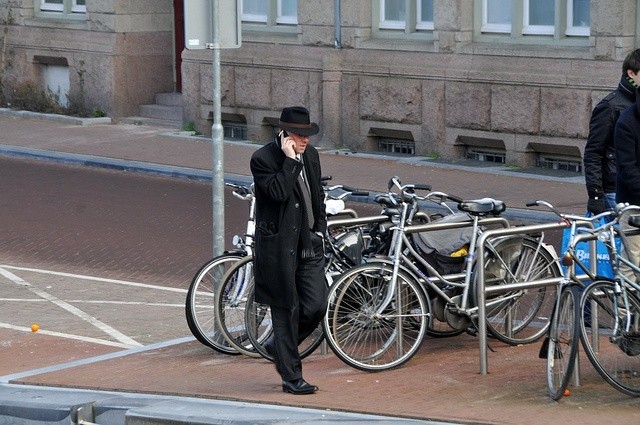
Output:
[271,107,319,136]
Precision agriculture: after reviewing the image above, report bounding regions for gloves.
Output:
[587,194,605,215]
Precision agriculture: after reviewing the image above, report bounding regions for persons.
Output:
[247,104,332,395]
[612,87,640,338]
[585,48,640,329]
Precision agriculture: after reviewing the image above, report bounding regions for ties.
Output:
[296,158,315,229]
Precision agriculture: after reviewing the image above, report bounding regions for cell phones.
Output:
[277,129,288,137]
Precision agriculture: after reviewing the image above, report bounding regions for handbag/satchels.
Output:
[408,210,483,275]
[560,211,622,291]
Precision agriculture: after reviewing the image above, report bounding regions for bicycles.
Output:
[322,176,567,372]
[185,174,549,364]
[573,204,639,398]
[525,192,639,399]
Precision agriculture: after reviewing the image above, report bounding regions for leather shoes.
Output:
[282,378,318,394]
[264,341,282,375]
